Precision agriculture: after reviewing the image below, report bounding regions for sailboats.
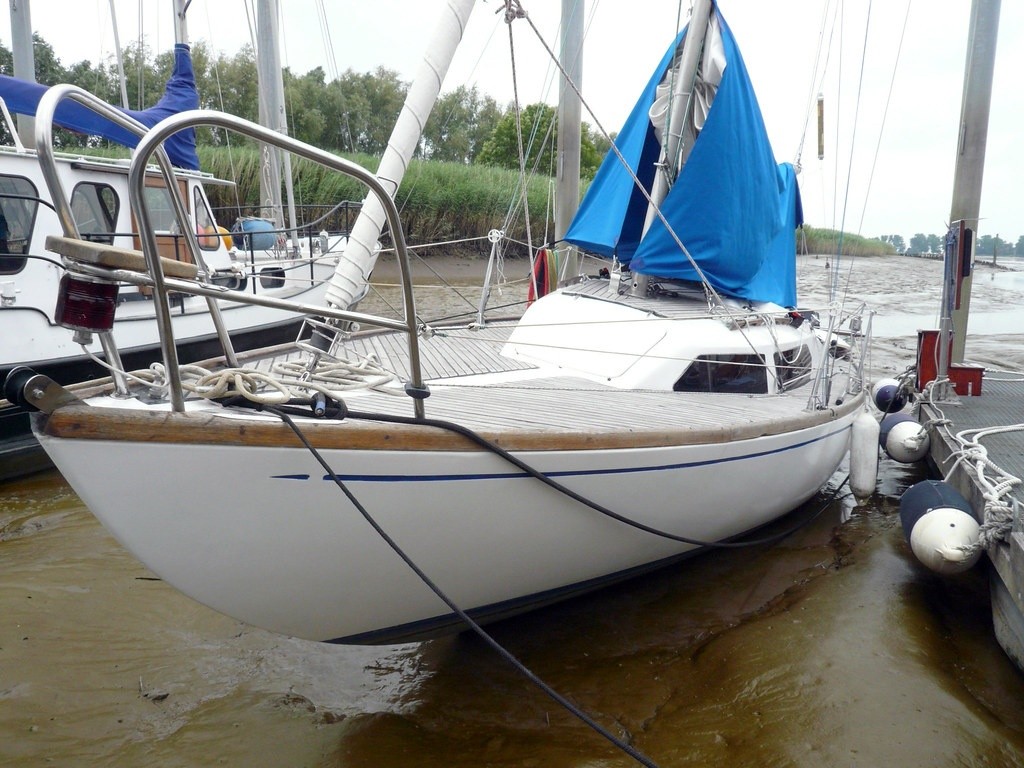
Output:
[0,0,372,486]
[871,0,1024,673]
[0,0,881,766]
